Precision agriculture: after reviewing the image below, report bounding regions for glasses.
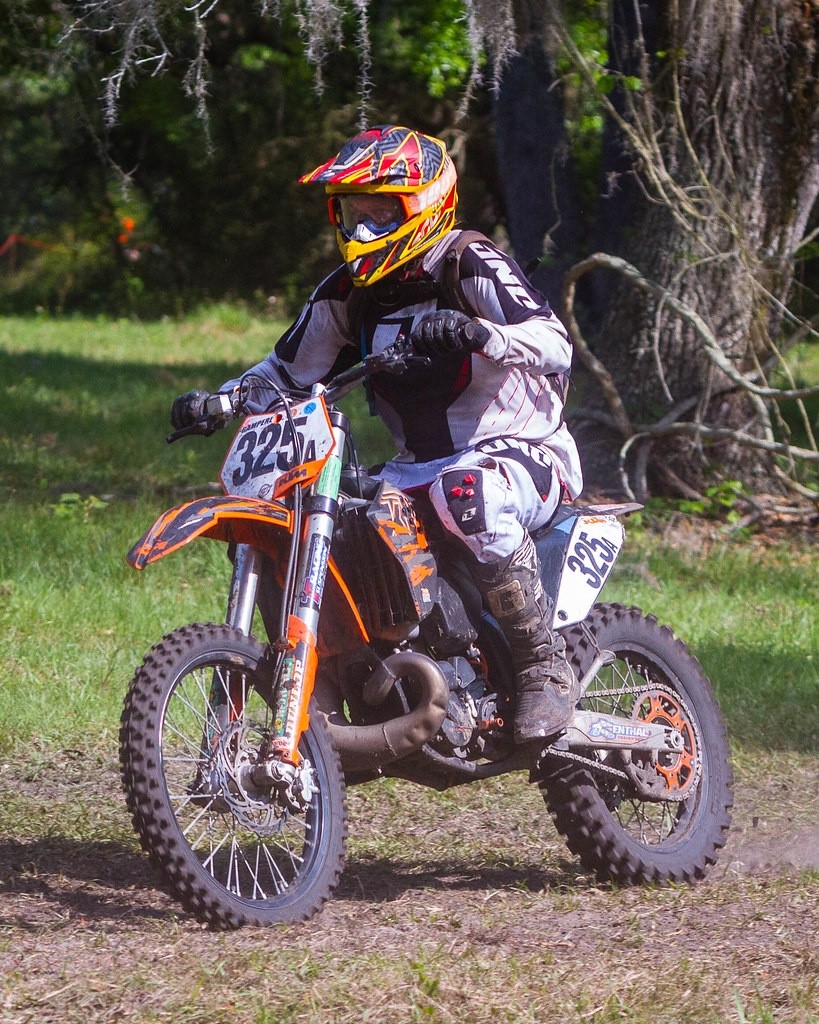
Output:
[340,197,403,231]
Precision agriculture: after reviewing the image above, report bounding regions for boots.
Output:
[467,526,581,744]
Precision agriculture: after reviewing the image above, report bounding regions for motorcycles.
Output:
[113,321,737,934]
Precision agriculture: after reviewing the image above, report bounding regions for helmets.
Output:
[297,125,458,288]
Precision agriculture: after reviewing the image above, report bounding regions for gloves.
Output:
[170,390,226,437]
[411,309,490,363]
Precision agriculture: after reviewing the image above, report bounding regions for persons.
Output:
[168,126,582,744]
